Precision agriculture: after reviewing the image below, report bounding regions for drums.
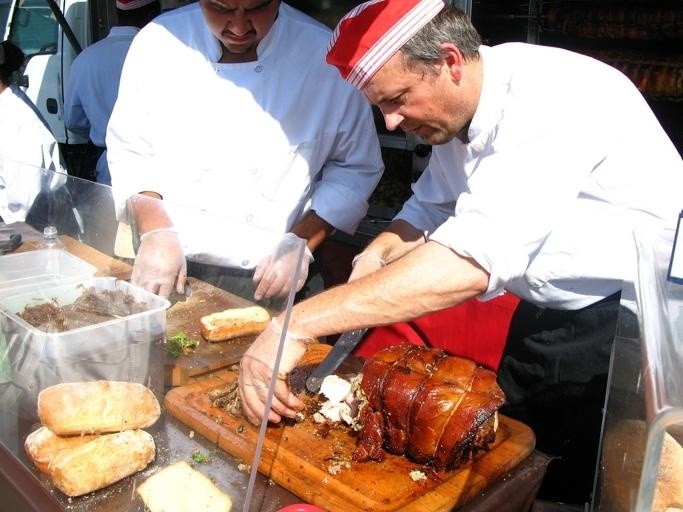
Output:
[0,235,22,255]
[306,329,373,394]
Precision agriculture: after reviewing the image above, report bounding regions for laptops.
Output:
[603,420,683,512]
[37,381,160,436]
[138,461,232,512]
[49,429,157,497]
[200,306,270,342]
[24,426,109,474]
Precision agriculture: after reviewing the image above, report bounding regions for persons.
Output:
[102,0,387,344]
[238,0,680,511]
[62,0,157,255]
[1,40,82,245]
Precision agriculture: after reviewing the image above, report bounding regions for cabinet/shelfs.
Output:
[40,226,68,275]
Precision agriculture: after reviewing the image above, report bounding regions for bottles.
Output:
[325,0,446,92]
